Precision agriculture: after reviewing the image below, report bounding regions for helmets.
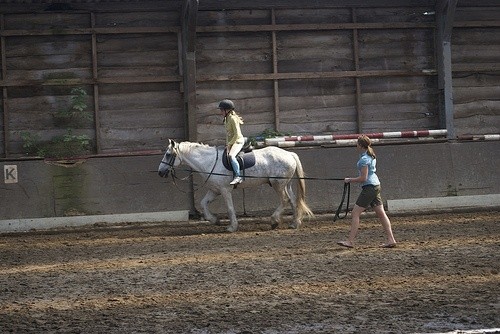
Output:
[217,100,235,110]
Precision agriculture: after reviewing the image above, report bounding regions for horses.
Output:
[158,138,315,231]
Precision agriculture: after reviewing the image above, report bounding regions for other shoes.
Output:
[380,243,397,248]
[337,242,355,249]
[230,178,243,184]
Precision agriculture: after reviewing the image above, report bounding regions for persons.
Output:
[336,135,397,248]
[217,100,245,185]
